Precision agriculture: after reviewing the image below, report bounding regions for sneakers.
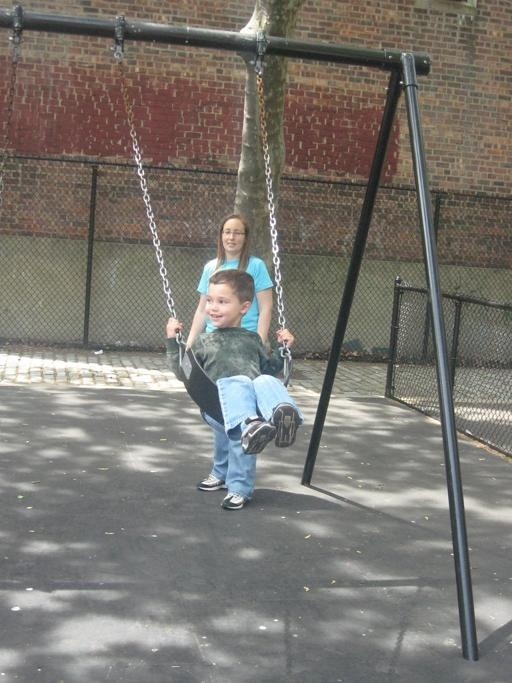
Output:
[199,474,226,491]
[221,493,249,509]
[271,403,300,448]
[241,420,277,455]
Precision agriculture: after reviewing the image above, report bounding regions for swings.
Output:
[113,40,294,426]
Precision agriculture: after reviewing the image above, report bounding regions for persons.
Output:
[166,269,304,454]
[185,214,275,512]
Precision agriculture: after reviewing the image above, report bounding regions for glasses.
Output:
[222,230,246,236]
